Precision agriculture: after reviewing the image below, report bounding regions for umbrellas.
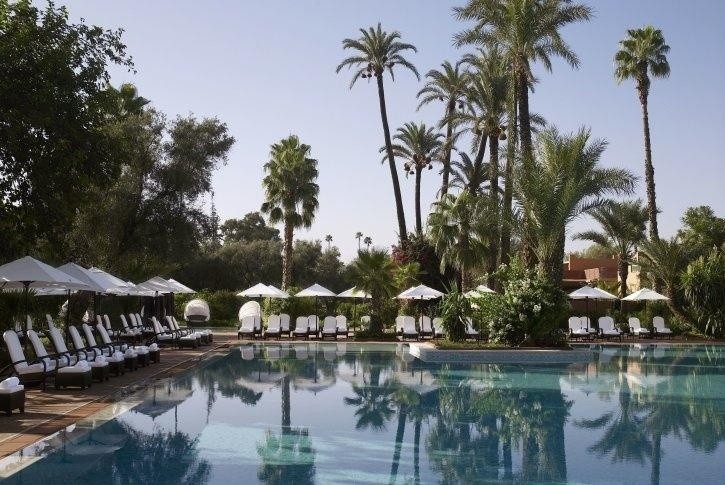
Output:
[621,288,671,319]
[573,287,618,329]
[235,283,288,308]
[336,286,372,332]
[237,368,500,401]
[392,281,497,339]
[566,285,609,331]
[58,380,196,457]
[623,357,672,389]
[294,283,337,333]
[576,360,609,396]
[0,256,198,352]
[249,285,290,308]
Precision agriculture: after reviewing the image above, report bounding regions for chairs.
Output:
[1,312,212,420]
[239,344,347,363]
[235,312,370,342]
[393,344,418,363]
[571,343,666,363]
[393,315,480,343]
[567,314,674,341]
